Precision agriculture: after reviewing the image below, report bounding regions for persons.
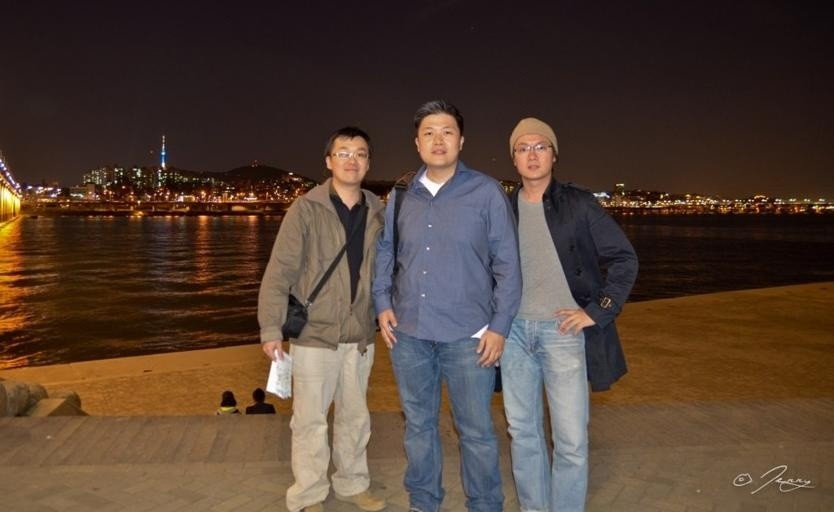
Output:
[215,389,241,414]
[244,387,276,414]
[497,116,641,512]
[372,97,521,512]
[256,124,385,510]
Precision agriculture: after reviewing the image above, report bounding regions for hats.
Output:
[509,117,558,158]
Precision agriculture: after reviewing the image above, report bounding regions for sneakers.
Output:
[336,491,389,512]
[303,504,323,512]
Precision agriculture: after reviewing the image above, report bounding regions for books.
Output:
[263,348,293,400]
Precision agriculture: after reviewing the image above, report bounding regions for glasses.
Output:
[333,151,369,161]
[513,144,554,154]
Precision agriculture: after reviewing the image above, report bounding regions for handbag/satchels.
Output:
[280,294,310,341]
